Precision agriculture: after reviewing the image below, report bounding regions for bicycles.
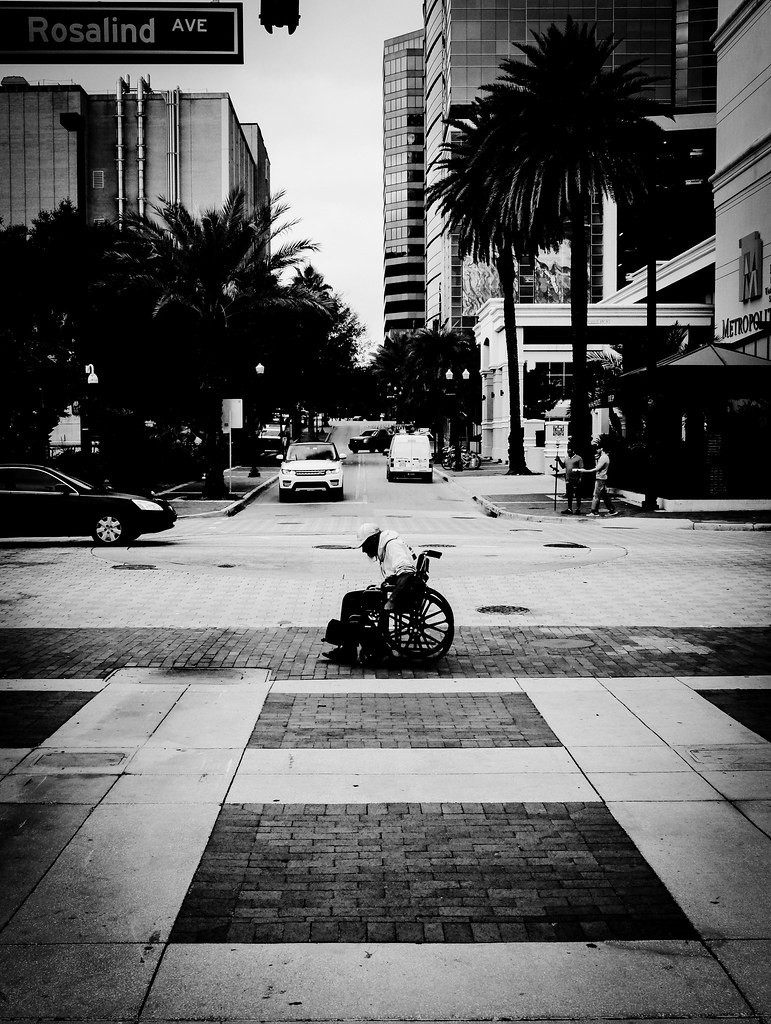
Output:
[439,445,483,472]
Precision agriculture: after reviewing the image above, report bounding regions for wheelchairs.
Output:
[320,548,455,673]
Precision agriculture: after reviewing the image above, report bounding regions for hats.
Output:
[353,524,382,548]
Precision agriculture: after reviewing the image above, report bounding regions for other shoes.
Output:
[604,510,618,516]
[561,509,573,514]
[586,512,599,517]
[322,648,357,664]
[573,510,582,515]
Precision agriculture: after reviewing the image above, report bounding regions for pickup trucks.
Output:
[347,429,395,453]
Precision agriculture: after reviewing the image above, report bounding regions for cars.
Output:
[0,461,179,549]
[383,433,435,484]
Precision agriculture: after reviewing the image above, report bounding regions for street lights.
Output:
[244,362,266,481]
[444,368,471,472]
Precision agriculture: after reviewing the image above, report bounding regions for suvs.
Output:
[275,440,349,502]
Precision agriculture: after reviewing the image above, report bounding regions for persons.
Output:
[556,448,583,515]
[322,521,416,668]
[579,445,618,517]
